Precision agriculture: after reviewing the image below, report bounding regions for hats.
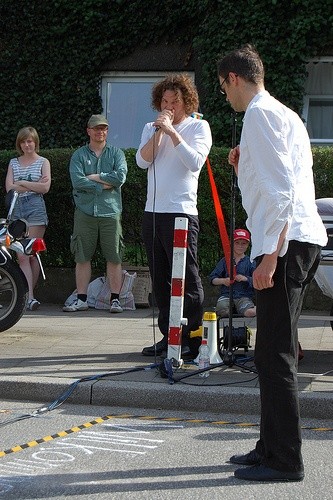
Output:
[88,115,109,127]
[233,229,250,242]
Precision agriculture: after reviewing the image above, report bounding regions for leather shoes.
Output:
[142,337,168,356]
[161,346,197,360]
[230,450,305,480]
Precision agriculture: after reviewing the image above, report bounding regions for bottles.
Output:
[198,340,211,377]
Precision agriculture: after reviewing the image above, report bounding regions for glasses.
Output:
[219,75,239,95]
[89,128,107,131]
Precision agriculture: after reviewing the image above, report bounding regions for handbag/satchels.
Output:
[65,270,137,310]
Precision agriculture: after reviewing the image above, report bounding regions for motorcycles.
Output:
[0,217,46,333]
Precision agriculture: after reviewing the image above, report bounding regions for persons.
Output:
[208,228,256,319]
[217,44,328,481]
[63,115,128,314]
[5,127,51,311]
[137,73,212,362]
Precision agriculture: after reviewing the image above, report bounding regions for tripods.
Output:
[169,108,261,385]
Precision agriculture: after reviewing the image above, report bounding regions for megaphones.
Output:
[193,312,223,365]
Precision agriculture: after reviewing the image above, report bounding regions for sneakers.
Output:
[27,299,40,310]
[63,299,89,312]
[110,299,123,313]
